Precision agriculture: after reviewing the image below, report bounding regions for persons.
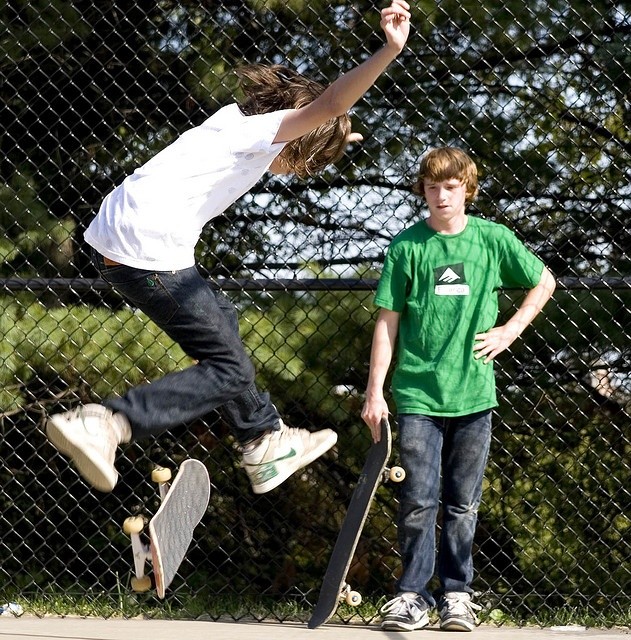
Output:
[42,0,414,497]
[360,145,558,633]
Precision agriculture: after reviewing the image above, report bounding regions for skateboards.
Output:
[308,416,406,629]
[123,459,211,599]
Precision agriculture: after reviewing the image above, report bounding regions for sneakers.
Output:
[438,590,482,631]
[45,403,134,494]
[243,418,339,496]
[379,590,430,631]
